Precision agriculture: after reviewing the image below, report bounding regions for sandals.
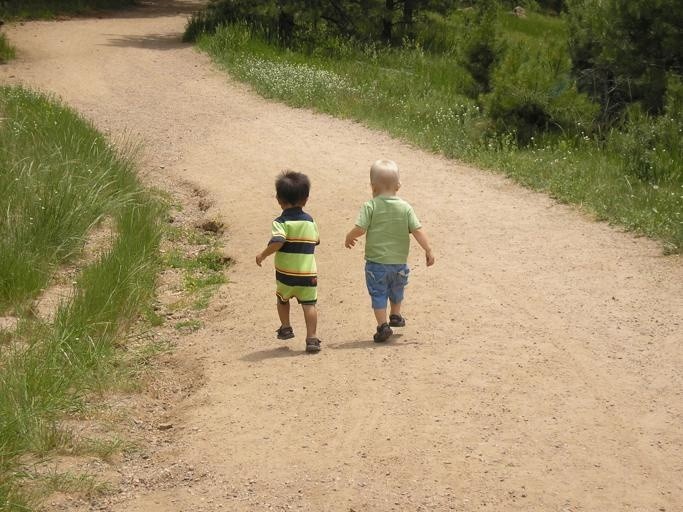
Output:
[374,323,392,341]
[277,326,294,339]
[306,337,321,351]
[389,315,405,327]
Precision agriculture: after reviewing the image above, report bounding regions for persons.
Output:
[256,170,321,353]
[345,159,434,342]
